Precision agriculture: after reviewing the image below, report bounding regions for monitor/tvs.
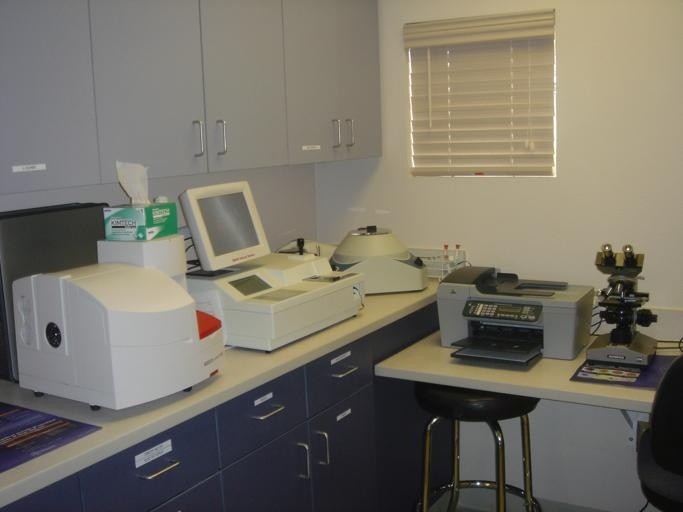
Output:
[179,181,271,272]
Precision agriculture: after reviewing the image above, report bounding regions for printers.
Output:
[436,266,593,369]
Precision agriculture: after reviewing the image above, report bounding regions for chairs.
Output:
[637,354,681,511]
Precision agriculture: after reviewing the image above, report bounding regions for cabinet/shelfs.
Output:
[281,1,384,166]
[214,327,380,510]
[0,406,218,512]
[89,1,287,188]
[0,1,101,195]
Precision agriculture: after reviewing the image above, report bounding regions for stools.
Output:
[413,380,539,512]
